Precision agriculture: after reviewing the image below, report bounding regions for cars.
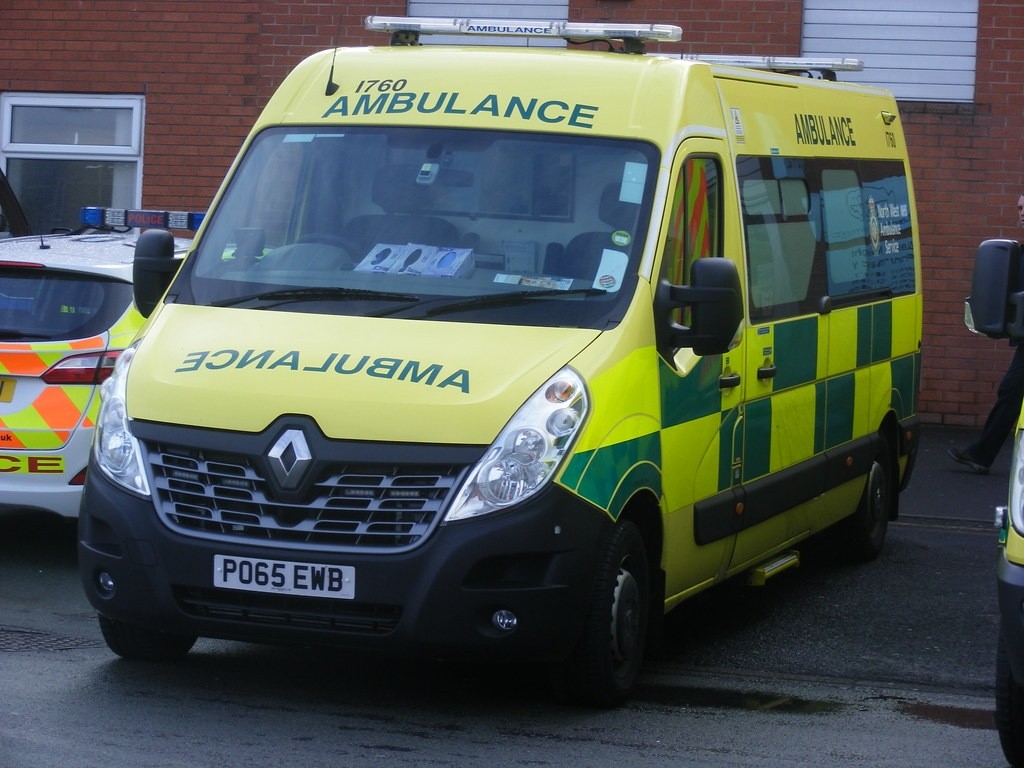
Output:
[0,203,283,522]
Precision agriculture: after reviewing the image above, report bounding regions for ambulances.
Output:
[962,239,1024,768]
[76,14,925,714]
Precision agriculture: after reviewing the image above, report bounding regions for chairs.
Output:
[341,163,460,262]
[555,182,641,281]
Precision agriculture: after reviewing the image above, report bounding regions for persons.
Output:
[947,191,1024,473]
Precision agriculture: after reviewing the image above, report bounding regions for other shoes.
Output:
[947,447,990,474]
